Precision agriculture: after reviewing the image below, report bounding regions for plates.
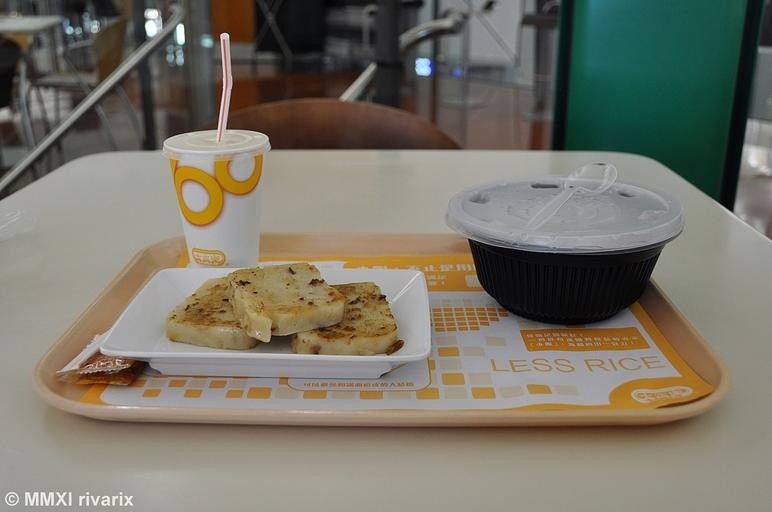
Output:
[99,266,434,381]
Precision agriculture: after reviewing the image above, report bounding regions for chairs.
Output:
[208,99,462,149]
[0,16,143,181]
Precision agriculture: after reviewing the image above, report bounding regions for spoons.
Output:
[505,160,619,247]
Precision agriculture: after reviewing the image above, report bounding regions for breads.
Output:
[166,263,404,356]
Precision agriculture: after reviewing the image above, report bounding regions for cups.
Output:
[163,127,273,273]
